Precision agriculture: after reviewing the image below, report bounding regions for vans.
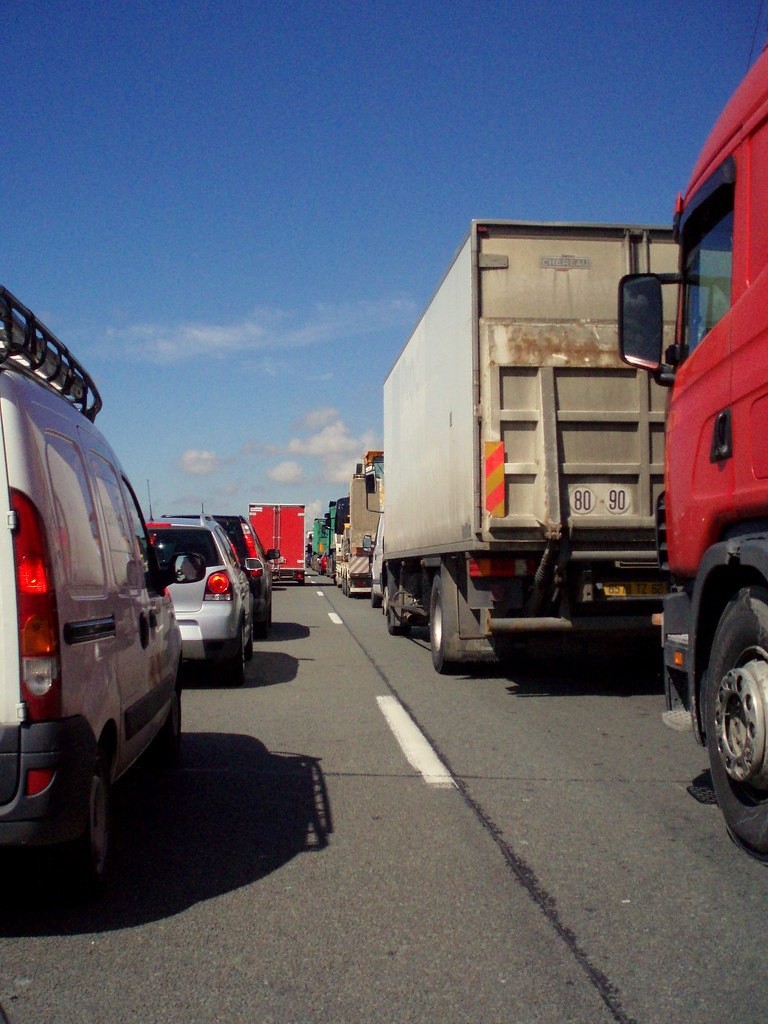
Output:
[0,285,208,882]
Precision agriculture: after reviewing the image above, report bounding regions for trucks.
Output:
[364,217,737,675]
[248,503,307,586]
[306,449,384,599]
[616,41,768,867]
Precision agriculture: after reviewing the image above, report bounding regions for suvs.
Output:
[160,514,280,639]
[143,513,265,687]
